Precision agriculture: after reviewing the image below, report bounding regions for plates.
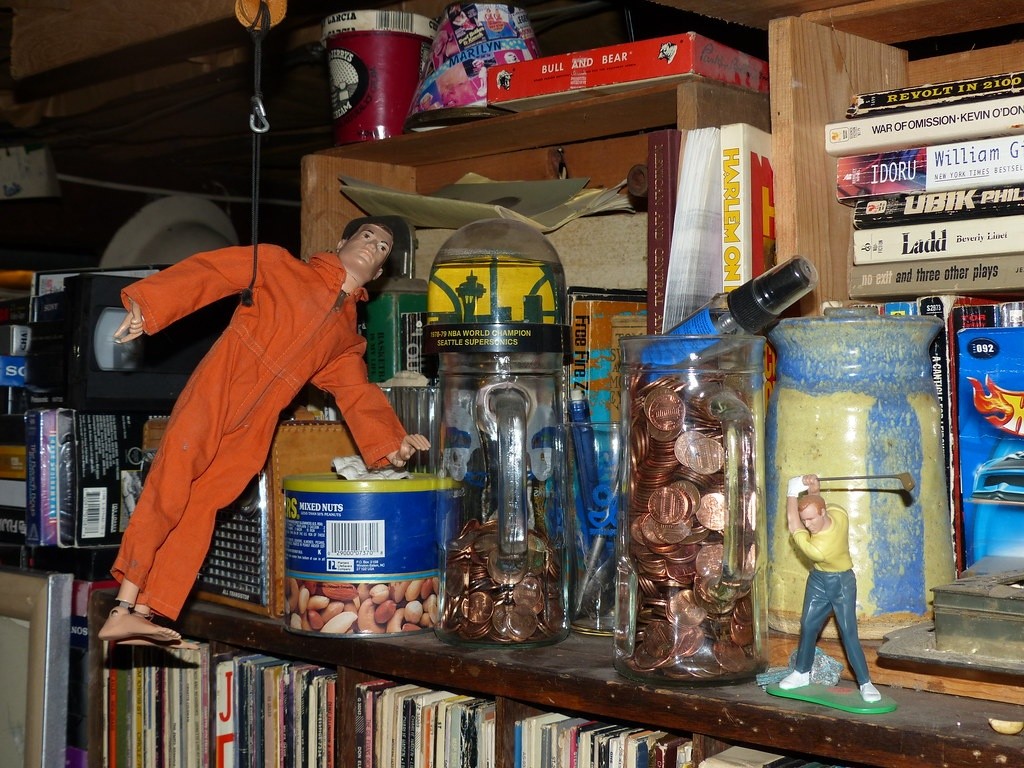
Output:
[98,195,238,269]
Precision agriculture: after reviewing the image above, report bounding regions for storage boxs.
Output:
[487,31,769,113]
[25,406,146,549]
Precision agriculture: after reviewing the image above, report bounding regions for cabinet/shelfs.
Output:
[84,0,1024,767]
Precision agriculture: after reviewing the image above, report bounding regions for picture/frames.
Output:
[0,565,74,768]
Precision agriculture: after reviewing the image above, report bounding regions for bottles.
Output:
[631,253,818,378]
[760,313,956,641]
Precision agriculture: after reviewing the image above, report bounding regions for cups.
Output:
[322,10,441,145]
[616,331,770,691]
[421,323,572,650]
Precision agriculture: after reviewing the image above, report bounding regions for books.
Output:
[660,126,723,337]
[340,166,635,233]
[822,71,1024,571]
[99,633,691,768]
[724,122,775,312]
[0,261,160,579]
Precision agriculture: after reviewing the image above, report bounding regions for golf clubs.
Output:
[816,472,915,492]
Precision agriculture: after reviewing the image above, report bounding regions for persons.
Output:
[779,474,882,703]
[98,214,430,646]
